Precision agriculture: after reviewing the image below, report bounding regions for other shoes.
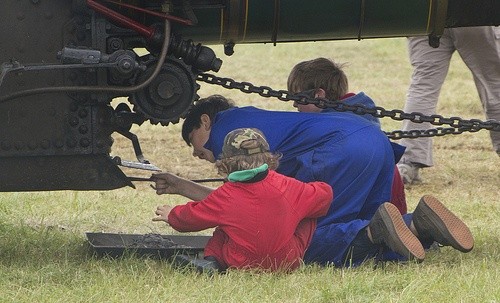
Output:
[398,162,418,183]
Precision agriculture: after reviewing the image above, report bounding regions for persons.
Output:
[150,58,475,275]
[396,26,500,184]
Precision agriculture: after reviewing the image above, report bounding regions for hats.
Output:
[222,129,270,157]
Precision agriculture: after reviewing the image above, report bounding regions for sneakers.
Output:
[414,195,474,252]
[371,202,424,262]
